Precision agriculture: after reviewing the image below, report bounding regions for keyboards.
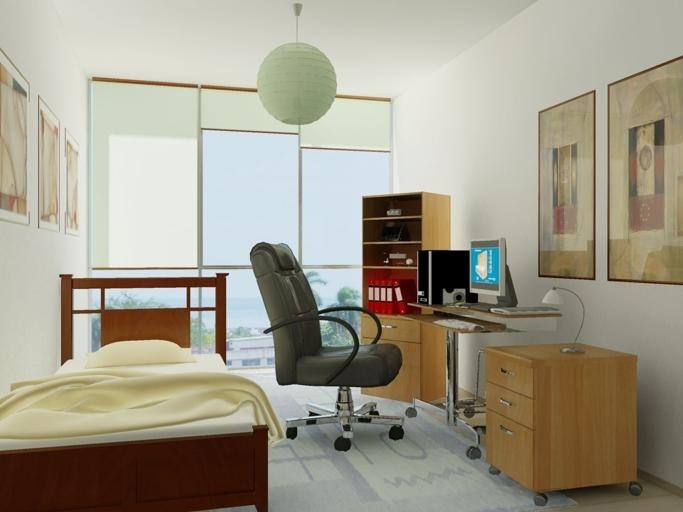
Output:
[433,319,480,329]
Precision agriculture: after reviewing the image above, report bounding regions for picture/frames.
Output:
[0,48,31,225]
[607,54,683,285]
[65,128,80,236]
[38,94,61,233]
[537,90,596,280]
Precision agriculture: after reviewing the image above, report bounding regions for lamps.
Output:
[541,286,585,354]
[257,0,338,126]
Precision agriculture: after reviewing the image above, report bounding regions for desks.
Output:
[406,303,562,460]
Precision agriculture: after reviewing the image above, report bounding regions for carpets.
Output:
[203,372,578,512]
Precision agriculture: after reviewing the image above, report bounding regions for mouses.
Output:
[469,325,484,331]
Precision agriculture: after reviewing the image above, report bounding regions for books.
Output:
[366,276,416,316]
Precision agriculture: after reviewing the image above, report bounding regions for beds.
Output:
[0,273,269,512]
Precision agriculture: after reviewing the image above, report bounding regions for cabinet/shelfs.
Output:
[360,192,451,404]
[485,344,643,506]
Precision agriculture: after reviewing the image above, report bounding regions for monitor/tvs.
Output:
[469,238,518,312]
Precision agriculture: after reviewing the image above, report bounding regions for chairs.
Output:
[250,242,404,451]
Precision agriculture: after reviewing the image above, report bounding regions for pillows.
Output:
[84,339,197,368]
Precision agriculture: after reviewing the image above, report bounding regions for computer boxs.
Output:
[417,249,478,306]
